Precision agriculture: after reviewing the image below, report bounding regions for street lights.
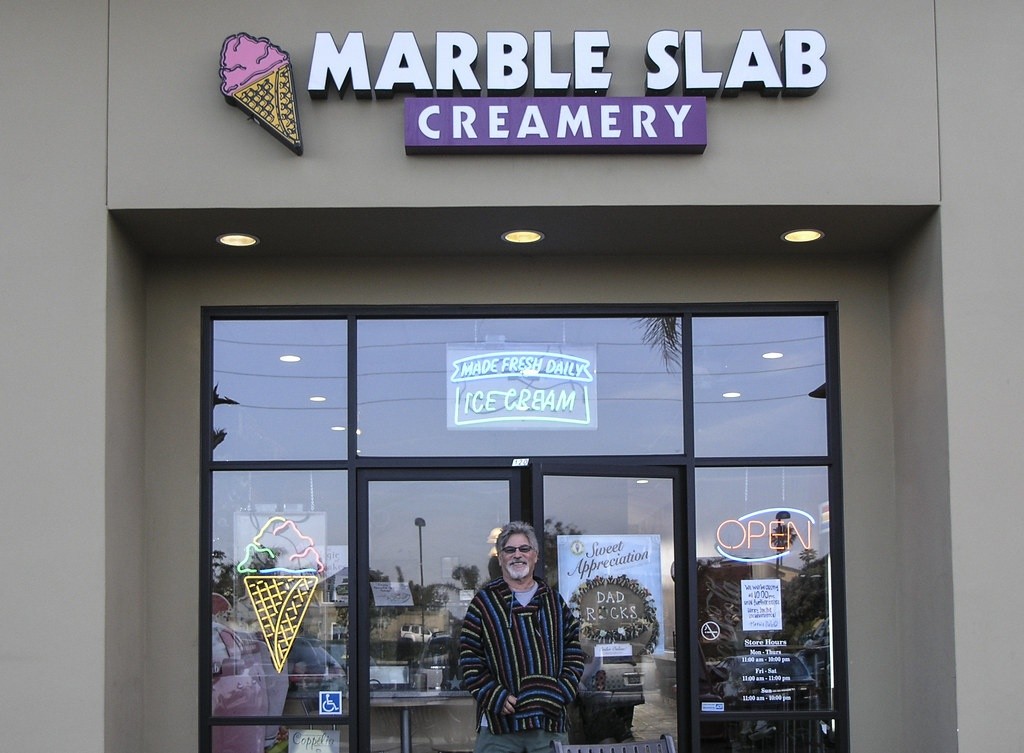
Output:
[414,516,428,648]
[774,512,792,579]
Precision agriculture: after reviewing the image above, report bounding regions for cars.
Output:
[400,625,433,643]
[212,617,830,753]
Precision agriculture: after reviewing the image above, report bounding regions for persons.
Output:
[458,521,587,753]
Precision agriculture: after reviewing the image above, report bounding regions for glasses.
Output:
[497,544,534,554]
